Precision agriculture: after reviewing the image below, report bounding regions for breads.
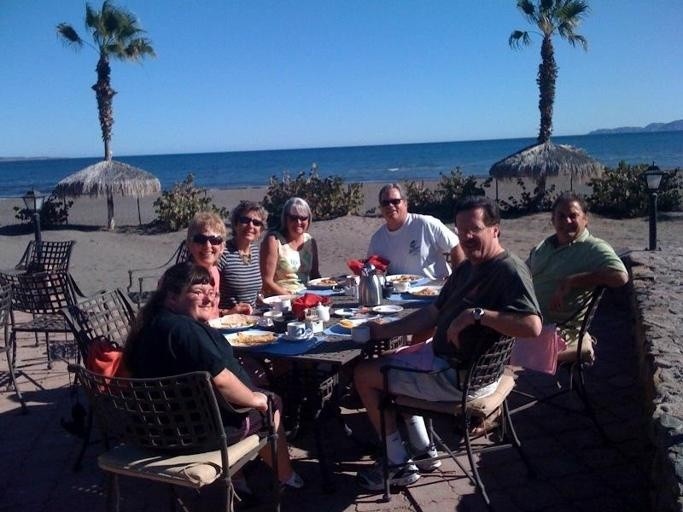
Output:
[414,287,437,297]
[317,277,338,284]
[235,333,274,344]
[340,319,352,327]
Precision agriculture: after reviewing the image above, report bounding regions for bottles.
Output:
[313,302,329,323]
[351,280,359,300]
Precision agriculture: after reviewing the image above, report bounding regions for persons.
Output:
[259,197,321,295]
[523,191,628,345]
[185,210,253,320]
[352,196,545,491]
[367,182,465,344]
[215,200,268,311]
[122,263,309,497]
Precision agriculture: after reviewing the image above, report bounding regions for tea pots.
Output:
[358,262,384,309]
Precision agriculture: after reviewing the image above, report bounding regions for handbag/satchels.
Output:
[88,337,123,389]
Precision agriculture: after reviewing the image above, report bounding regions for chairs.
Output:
[510,284,608,432]
[68,365,280,512]
[378,332,522,507]
[1,240,190,470]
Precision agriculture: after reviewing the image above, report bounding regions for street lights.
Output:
[640,161,662,250]
[21,189,45,260]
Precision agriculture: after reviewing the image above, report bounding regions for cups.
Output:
[262,310,283,331]
[280,298,293,312]
[345,275,359,288]
[350,326,370,344]
[392,280,408,294]
[284,321,306,339]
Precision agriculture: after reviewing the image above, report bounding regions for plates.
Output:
[372,304,403,315]
[307,275,358,289]
[334,307,364,317]
[262,294,299,306]
[221,332,278,349]
[385,274,424,284]
[407,285,444,298]
[206,312,261,332]
[337,317,366,329]
[281,330,314,341]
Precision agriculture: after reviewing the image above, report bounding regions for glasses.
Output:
[287,213,309,221]
[240,216,263,226]
[192,234,223,245]
[381,198,401,207]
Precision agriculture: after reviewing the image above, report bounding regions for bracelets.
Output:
[220,307,224,317]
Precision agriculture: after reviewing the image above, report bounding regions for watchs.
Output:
[471,305,485,324]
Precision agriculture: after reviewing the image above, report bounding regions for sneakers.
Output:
[358,454,420,489]
[408,444,441,473]
